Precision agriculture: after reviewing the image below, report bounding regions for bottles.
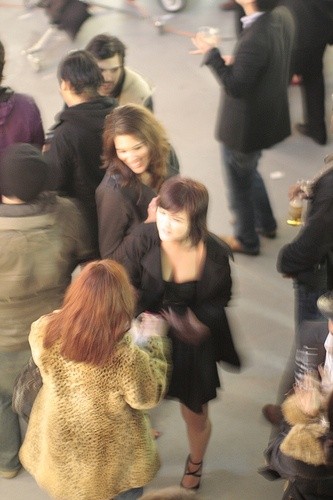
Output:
[286,178,304,225]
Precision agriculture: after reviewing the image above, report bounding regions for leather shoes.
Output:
[264,404,283,425]
[230,219,277,238]
[296,123,326,144]
[220,235,260,256]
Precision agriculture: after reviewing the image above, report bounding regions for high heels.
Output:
[180,454,203,491]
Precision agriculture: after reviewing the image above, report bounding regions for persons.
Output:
[18,259,168,500]
[41,51,120,262]
[262,153,333,423]
[95,104,181,266]
[0,40,45,157]
[289,0,333,145]
[0,143,98,478]
[121,178,243,491]
[263,291,333,500]
[189,0,296,255]
[42,34,153,157]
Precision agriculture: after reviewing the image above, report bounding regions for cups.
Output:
[198,27,217,46]
